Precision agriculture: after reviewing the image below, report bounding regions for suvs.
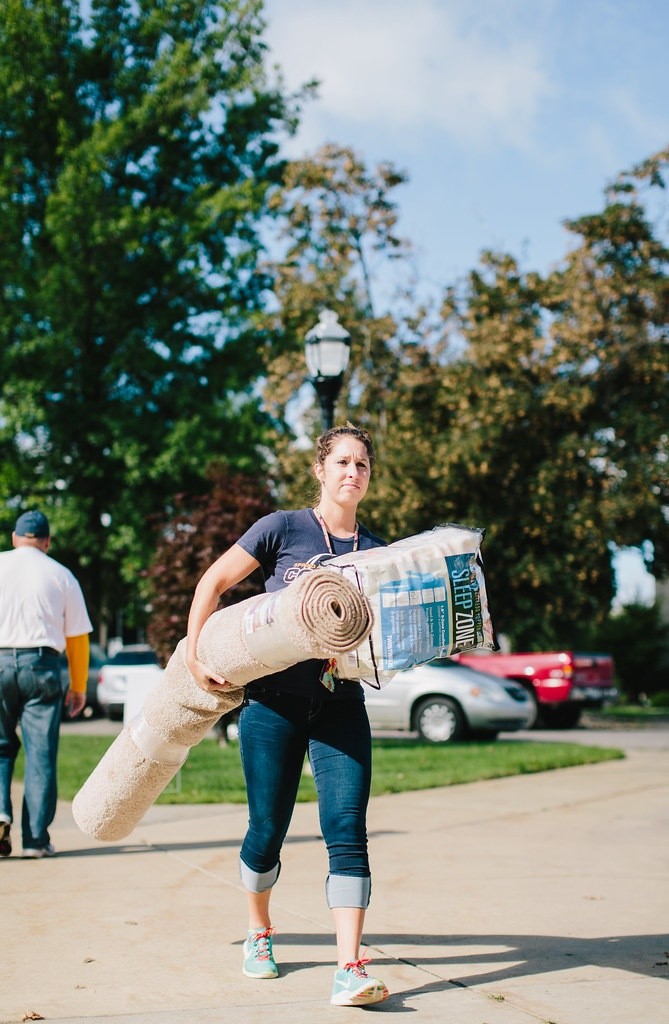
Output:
[96,643,165,723]
[56,643,105,722]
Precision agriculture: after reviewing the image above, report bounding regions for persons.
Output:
[1,510,93,858]
[184,428,393,1012]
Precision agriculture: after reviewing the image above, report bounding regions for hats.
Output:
[15,510,50,539]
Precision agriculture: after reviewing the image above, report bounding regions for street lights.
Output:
[303,307,350,434]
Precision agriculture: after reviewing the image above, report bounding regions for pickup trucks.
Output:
[454,638,617,734]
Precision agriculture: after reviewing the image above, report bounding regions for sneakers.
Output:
[21,843,54,858]
[242,929,278,978]
[330,958,388,1005]
[0,823,11,856]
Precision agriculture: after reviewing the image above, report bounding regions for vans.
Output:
[358,656,539,746]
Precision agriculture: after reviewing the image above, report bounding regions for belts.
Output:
[0,647,57,654]
